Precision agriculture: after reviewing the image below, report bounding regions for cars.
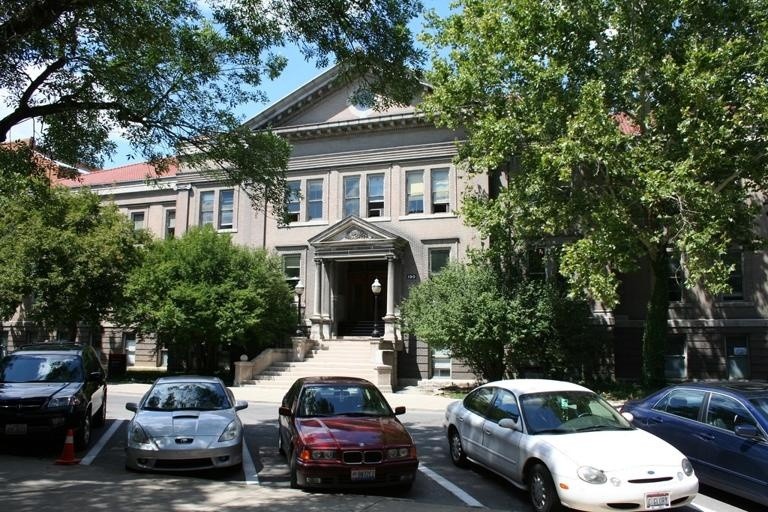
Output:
[277,376,419,490]
[125,375,248,472]
[444,381,700,510]
[620,382,767,507]
[0,342,107,447]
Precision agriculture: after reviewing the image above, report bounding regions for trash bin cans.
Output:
[109,354,126,377]
[291,336,307,361]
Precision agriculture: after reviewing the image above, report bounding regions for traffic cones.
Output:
[54,427,82,465]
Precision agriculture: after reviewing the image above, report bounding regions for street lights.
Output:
[371,277,382,338]
[294,280,304,337]
